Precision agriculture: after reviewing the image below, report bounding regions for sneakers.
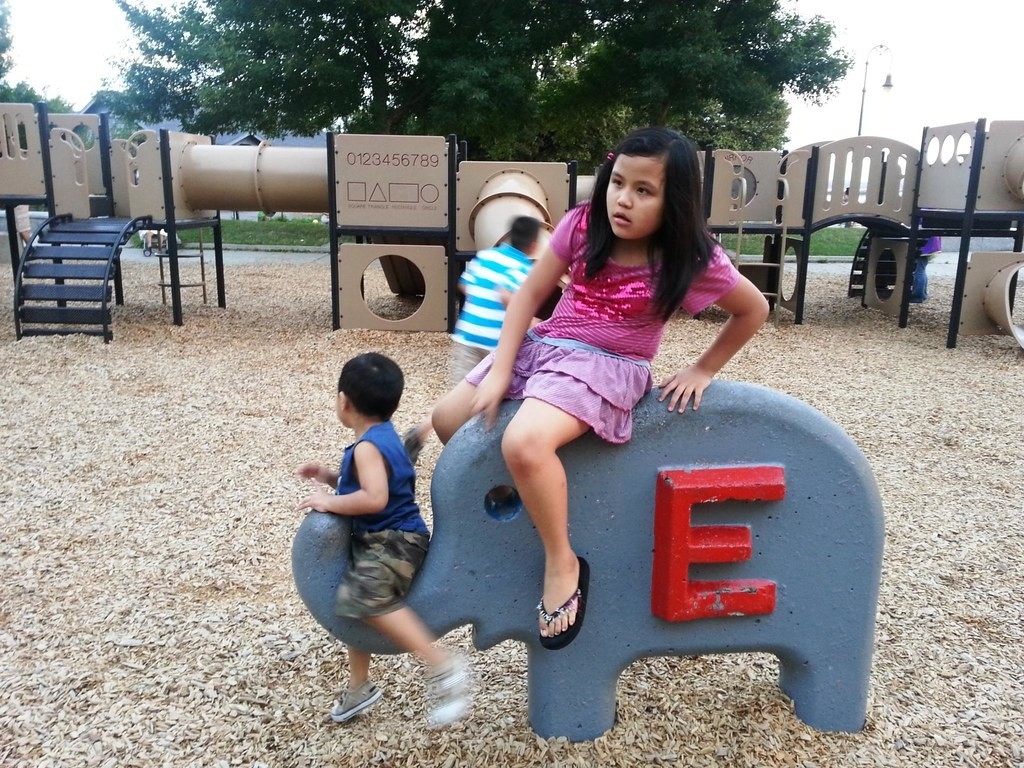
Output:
[331,680,382,723]
[428,659,469,726]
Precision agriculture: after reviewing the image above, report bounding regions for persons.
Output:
[12,205,32,242]
[430,125,770,650]
[446,214,539,396]
[911,234,944,303]
[844,186,851,228]
[137,229,167,251]
[295,351,472,731]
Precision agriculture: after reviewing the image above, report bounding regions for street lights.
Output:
[858,45,893,136]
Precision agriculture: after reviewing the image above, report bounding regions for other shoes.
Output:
[405,429,423,465]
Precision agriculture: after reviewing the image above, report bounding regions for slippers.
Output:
[537,556,589,650]
